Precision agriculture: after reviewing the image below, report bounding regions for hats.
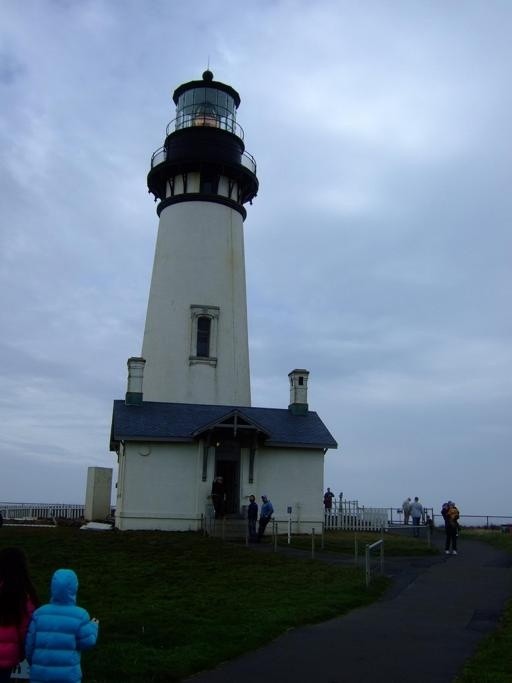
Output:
[449,503,455,507]
[262,495,267,498]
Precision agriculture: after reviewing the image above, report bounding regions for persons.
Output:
[448,502,460,536]
[210,474,227,521]
[402,496,411,526]
[323,487,335,515]
[256,495,274,539]
[441,501,459,555]
[247,494,259,541]
[410,495,424,537]
[0,544,43,682]
[24,566,100,683]
[424,515,433,535]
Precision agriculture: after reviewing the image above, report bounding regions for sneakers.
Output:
[444,550,450,555]
[452,550,457,556]
